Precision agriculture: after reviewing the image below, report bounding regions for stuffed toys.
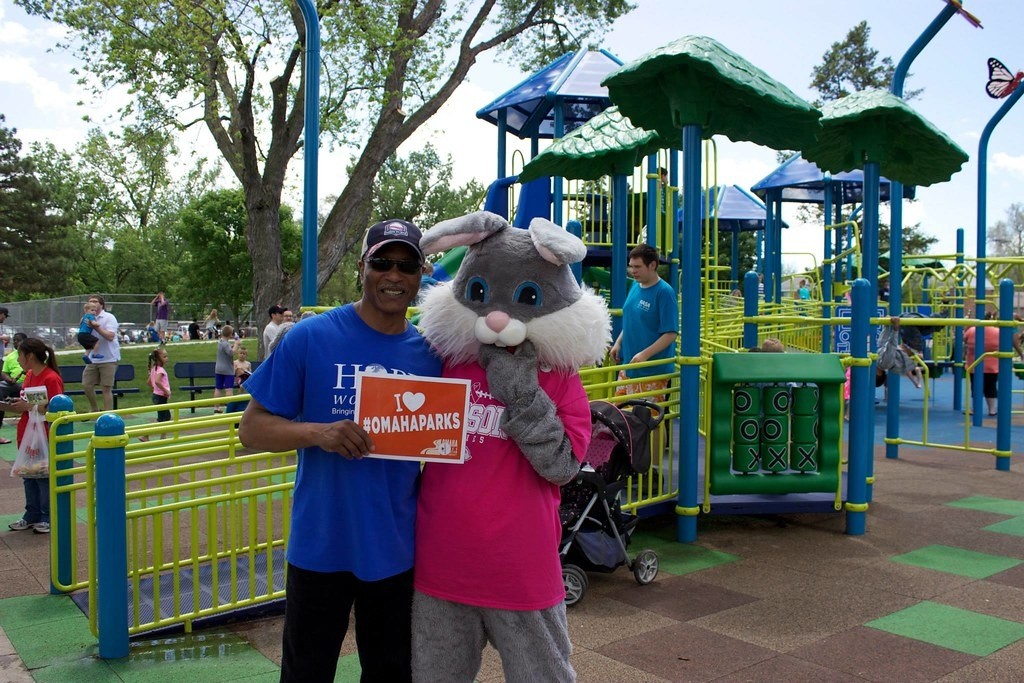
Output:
[416,210,614,683]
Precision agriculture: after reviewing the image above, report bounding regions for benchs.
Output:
[174,361,262,413]
[57,364,141,410]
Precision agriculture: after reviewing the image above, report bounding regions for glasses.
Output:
[364,258,422,274]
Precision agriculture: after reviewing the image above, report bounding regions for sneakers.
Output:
[8,519,36,529]
[33,522,49,533]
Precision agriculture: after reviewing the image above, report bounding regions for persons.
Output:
[0,307,12,377]
[151,292,168,346]
[234,347,251,394]
[301,311,317,319]
[8,338,64,533]
[203,309,219,339]
[213,325,242,413]
[226,320,230,325]
[280,309,298,324]
[172,333,182,341]
[0,332,26,429]
[263,304,287,359]
[239,218,450,683]
[138,349,171,442]
[182,330,190,340]
[657,167,1024,421]
[610,244,679,496]
[188,318,201,339]
[243,321,252,338]
[202,324,221,339]
[77,303,104,364]
[146,321,159,343]
[121,332,129,344]
[82,295,121,422]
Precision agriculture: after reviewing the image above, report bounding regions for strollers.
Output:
[557,398,665,607]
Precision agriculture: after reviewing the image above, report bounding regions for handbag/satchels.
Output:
[10,405,49,478]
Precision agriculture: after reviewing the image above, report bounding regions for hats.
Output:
[268,305,287,315]
[0,307,10,316]
[361,219,424,263]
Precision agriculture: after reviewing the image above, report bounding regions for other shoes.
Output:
[82,355,91,364]
[0,438,11,443]
[139,436,150,442]
[214,410,223,413]
[93,354,103,358]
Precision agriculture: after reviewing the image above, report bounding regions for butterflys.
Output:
[985,57,1024,99]
